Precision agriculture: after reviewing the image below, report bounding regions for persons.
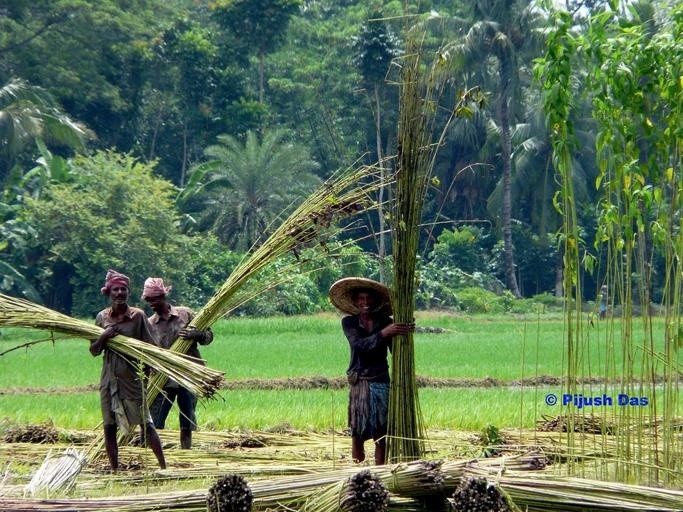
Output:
[328,276,415,465]
[139,276,214,449]
[88,268,168,470]
[599,285,607,320]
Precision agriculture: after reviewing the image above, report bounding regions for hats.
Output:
[329,277,391,315]
